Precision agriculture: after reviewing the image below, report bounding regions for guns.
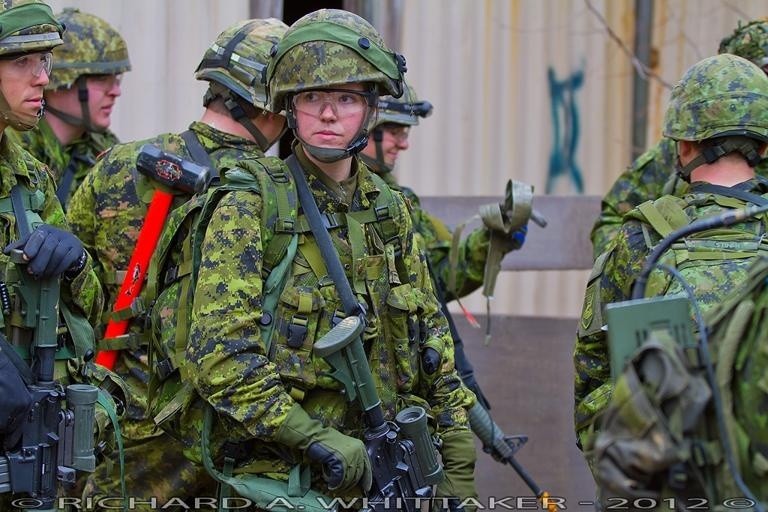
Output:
[467,401,560,512]
[312,316,445,511]
[0,246,100,512]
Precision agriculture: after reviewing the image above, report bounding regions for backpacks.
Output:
[142,148,304,488]
[622,190,768,342]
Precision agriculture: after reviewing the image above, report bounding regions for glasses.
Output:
[288,85,370,120]
[7,53,57,78]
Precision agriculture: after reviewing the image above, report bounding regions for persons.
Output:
[0,1,768,512]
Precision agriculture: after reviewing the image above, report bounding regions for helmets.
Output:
[660,52,768,143]
[0,0,64,57]
[42,9,133,94]
[718,20,768,70]
[266,6,408,115]
[363,75,421,137]
[193,15,291,117]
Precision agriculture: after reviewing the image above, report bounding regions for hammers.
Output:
[96,144,211,371]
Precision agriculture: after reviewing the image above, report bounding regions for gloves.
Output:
[0,349,35,452]
[2,222,87,281]
[431,431,482,512]
[484,201,532,254]
[273,400,376,495]
